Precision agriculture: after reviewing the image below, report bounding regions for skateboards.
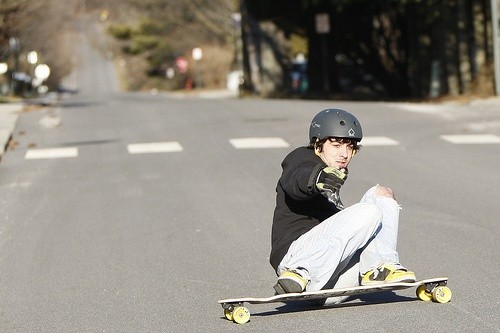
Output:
[217,277,452,324]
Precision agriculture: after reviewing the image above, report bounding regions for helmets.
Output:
[309,108,363,142]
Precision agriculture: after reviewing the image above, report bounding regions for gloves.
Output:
[316,167,349,193]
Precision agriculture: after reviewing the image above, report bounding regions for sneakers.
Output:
[273,269,308,295]
[361,262,416,287]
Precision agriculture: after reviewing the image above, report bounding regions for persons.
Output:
[269,109,417,293]
